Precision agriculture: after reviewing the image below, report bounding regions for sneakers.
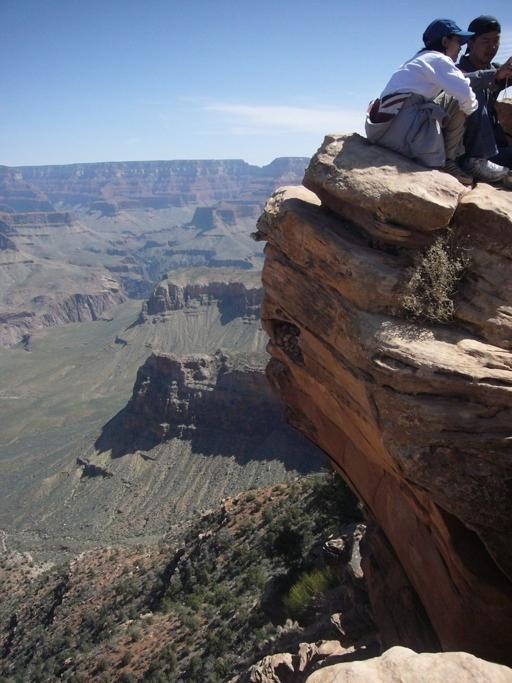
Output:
[439,159,474,185]
[460,156,512,190]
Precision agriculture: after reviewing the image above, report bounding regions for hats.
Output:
[464,14,500,55]
[422,18,475,45]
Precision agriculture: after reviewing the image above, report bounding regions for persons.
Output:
[365,16,512,184]
